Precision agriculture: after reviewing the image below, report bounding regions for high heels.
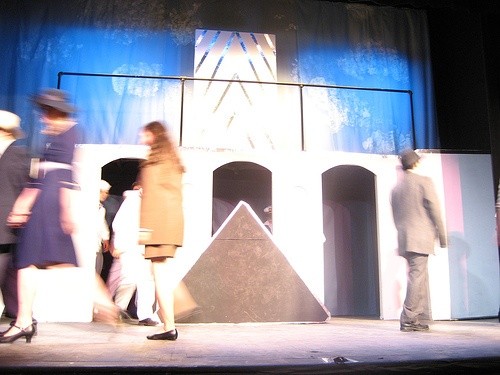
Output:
[0,323,35,344]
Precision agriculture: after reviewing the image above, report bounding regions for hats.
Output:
[0,109,21,130]
[101,179,111,190]
[36,87,74,113]
[400,149,420,164]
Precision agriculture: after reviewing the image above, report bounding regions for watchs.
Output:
[0,89,83,344]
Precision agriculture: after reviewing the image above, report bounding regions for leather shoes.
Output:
[146,329,178,340]
[400,321,429,332]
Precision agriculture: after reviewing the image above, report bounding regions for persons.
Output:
[0,110,28,325]
[141,121,184,339]
[495,183,500,250]
[95,179,112,290]
[109,181,154,328]
[391,149,447,331]
[104,182,145,306]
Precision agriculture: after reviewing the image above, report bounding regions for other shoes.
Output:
[0,317,37,337]
[138,318,156,326]
